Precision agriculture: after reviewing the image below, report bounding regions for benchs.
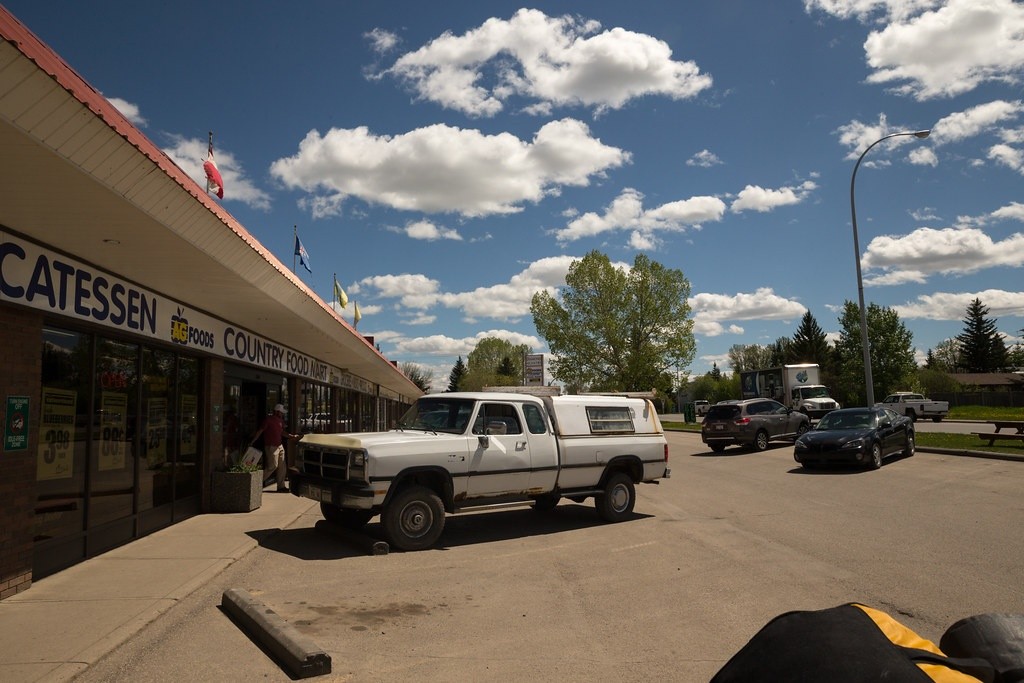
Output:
[970,432,1024,440]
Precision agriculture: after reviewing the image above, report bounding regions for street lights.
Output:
[851,127,931,406]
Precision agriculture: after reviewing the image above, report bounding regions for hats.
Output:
[274,404,288,414]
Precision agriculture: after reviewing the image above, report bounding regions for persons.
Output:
[250,405,299,493]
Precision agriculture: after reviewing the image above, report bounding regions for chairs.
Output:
[852,417,864,426]
[512,406,533,432]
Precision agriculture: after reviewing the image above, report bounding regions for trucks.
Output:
[740,364,841,418]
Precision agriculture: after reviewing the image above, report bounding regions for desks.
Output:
[987,420,1024,447]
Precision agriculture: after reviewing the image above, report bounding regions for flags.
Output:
[333,279,348,309]
[295,233,312,274]
[204,151,224,200]
[355,302,362,324]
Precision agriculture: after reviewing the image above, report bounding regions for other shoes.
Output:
[277,487,288,493]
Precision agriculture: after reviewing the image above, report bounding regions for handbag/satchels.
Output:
[709,602,1001,683]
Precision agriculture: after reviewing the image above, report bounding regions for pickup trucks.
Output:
[297,391,671,552]
[693,400,712,415]
[875,391,949,423]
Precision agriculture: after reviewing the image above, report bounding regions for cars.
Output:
[794,406,915,470]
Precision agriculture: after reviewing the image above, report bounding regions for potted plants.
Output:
[210,462,264,515]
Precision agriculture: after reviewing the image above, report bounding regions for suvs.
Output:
[701,397,810,453]
